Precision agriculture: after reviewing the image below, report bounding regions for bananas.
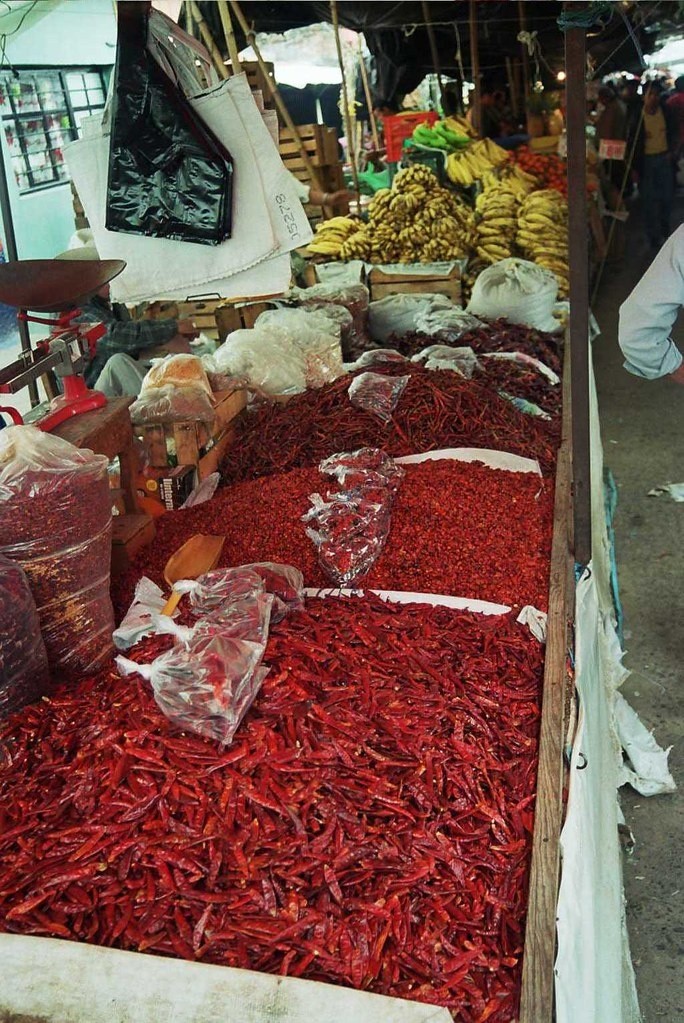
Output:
[513,187,571,300]
[302,109,538,305]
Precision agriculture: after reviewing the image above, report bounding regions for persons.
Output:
[365,99,400,162]
[617,223,684,388]
[50,226,200,395]
[287,168,359,207]
[586,67,683,247]
[441,83,515,142]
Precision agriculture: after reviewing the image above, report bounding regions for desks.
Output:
[9,395,138,511]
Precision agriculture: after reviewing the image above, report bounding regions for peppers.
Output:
[0,313,564,1022]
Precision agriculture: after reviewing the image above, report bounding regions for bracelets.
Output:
[323,192,329,205]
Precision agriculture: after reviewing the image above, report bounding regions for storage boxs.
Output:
[197,59,349,230]
[189,295,274,344]
[369,260,462,310]
[136,464,196,510]
[295,247,365,287]
[379,112,439,163]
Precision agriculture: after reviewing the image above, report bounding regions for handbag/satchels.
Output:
[60,0,314,308]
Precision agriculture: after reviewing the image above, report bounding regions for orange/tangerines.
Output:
[504,144,569,199]
